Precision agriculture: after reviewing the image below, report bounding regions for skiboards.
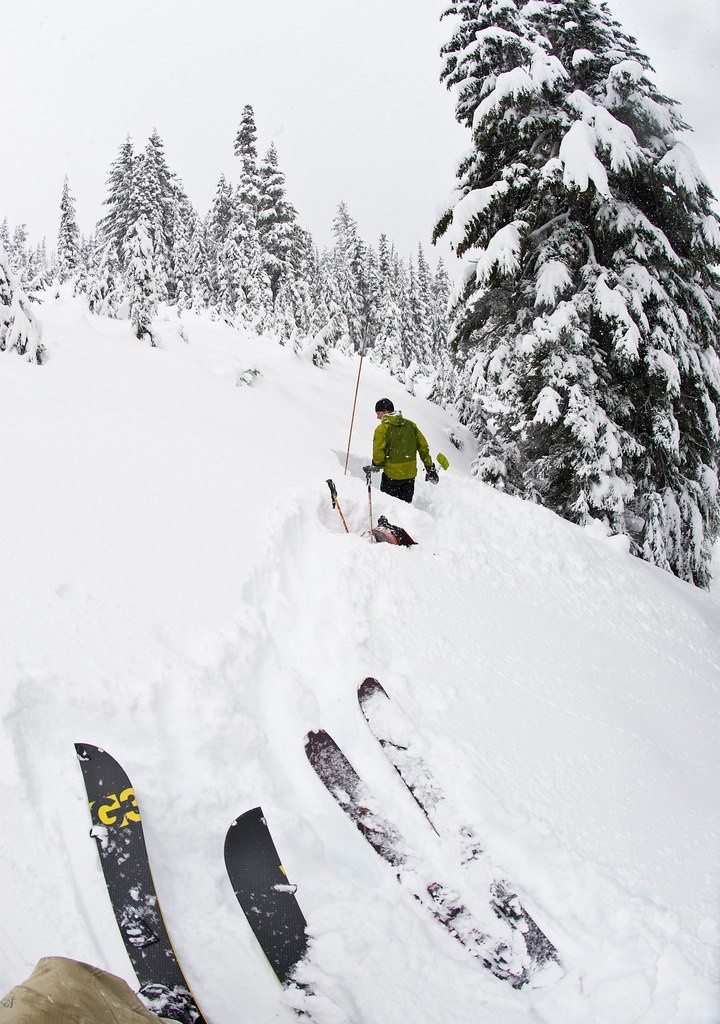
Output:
[305,676,571,990]
[75,743,315,1023]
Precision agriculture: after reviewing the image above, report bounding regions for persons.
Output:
[369,398,440,504]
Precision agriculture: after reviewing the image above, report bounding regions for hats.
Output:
[374,398,394,412]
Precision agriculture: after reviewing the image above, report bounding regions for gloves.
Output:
[363,465,380,473]
[425,463,439,484]
